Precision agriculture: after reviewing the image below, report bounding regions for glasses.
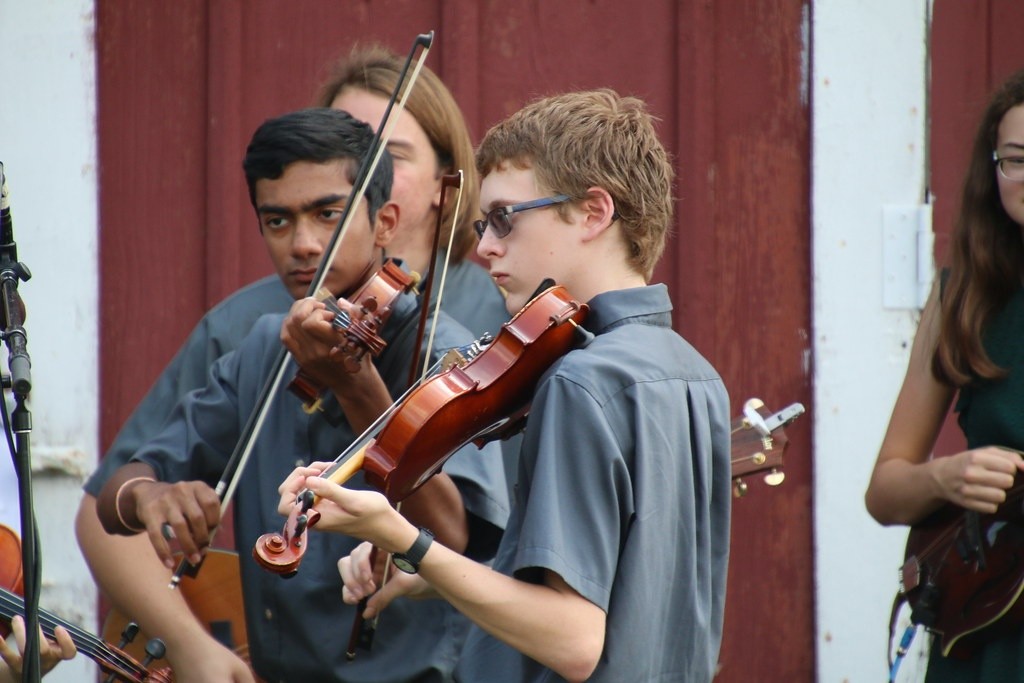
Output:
[473,192,620,242]
[992,150,1024,181]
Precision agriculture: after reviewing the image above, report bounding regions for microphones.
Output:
[0,162,27,331]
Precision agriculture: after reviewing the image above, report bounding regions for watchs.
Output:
[391,526,436,575]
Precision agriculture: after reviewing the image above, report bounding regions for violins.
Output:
[164,26,598,661]
[0,522,175,683]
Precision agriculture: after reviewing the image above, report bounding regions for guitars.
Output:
[899,451,1024,660]
[92,397,806,683]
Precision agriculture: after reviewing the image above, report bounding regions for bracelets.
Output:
[116,477,157,533]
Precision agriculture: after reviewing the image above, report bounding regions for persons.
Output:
[0,615,77,683]
[276,88,732,683]
[75,39,525,683]
[864,70,1023,682]
